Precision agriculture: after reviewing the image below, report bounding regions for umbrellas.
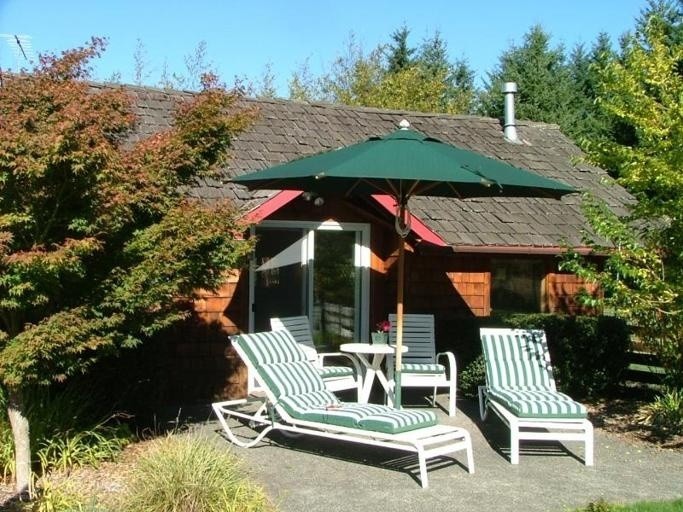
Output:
[219,116,582,412]
